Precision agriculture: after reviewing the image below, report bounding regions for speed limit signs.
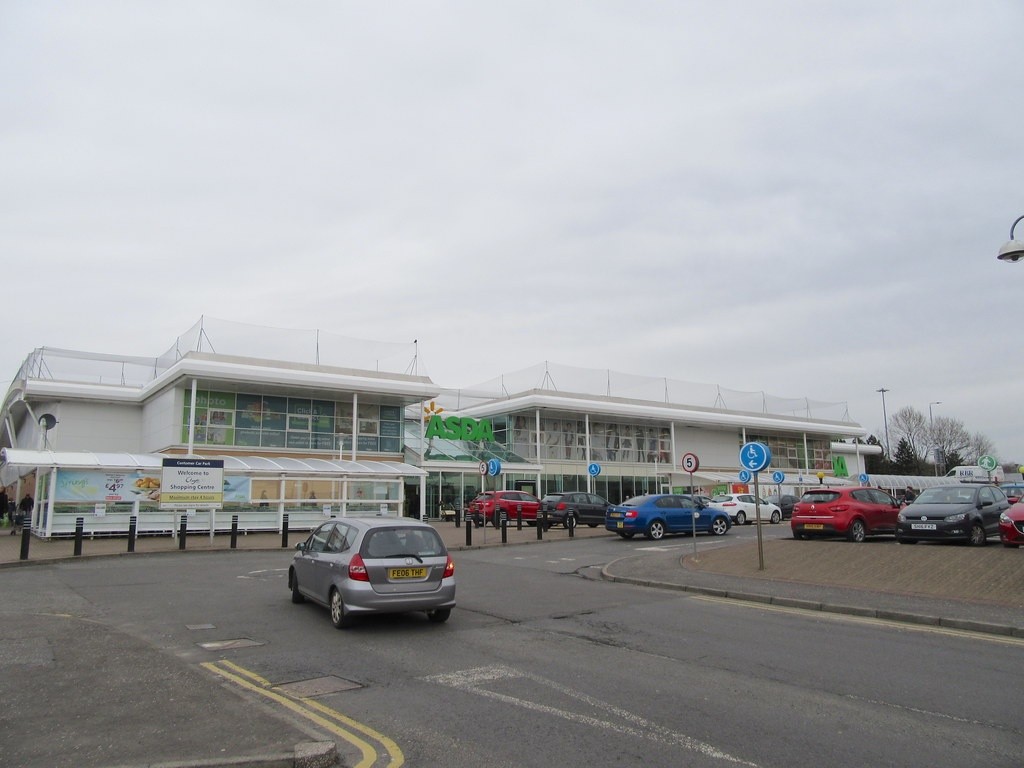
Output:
[479,461,488,474]
[682,453,699,473]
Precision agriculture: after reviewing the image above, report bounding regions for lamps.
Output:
[475,504,480,511]
[181,515,187,524]
[568,509,573,516]
[456,503,460,510]
[283,514,288,522]
[130,516,136,525]
[76,517,84,527]
[232,515,238,523]
[517,503,522,511]
[496,504,500,510]
[22,518,31,530]
[542,503,547,511]
[501,511,507,520]
[466,512,472,521]
[536,510,543,518]
[423,514,428,523]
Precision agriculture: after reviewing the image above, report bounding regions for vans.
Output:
[943,466,1005,484]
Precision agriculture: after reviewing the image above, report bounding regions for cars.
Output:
[605,493,782,541]
[761,495,800,520]
[897,482,1024,546]
[791,486,906,543]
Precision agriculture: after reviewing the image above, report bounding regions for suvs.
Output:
[469,490,542,527]
[289,519,456,629]
[539,492,616,528]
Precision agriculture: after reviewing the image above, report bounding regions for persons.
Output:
[904,486,915,506]
[0,486,35,535]
[355,488,362,505]
[308,491,319,505]
[260,490,269,507]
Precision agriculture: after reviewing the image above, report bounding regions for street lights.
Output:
[929,401,941,476]
[876,389,891,463]
